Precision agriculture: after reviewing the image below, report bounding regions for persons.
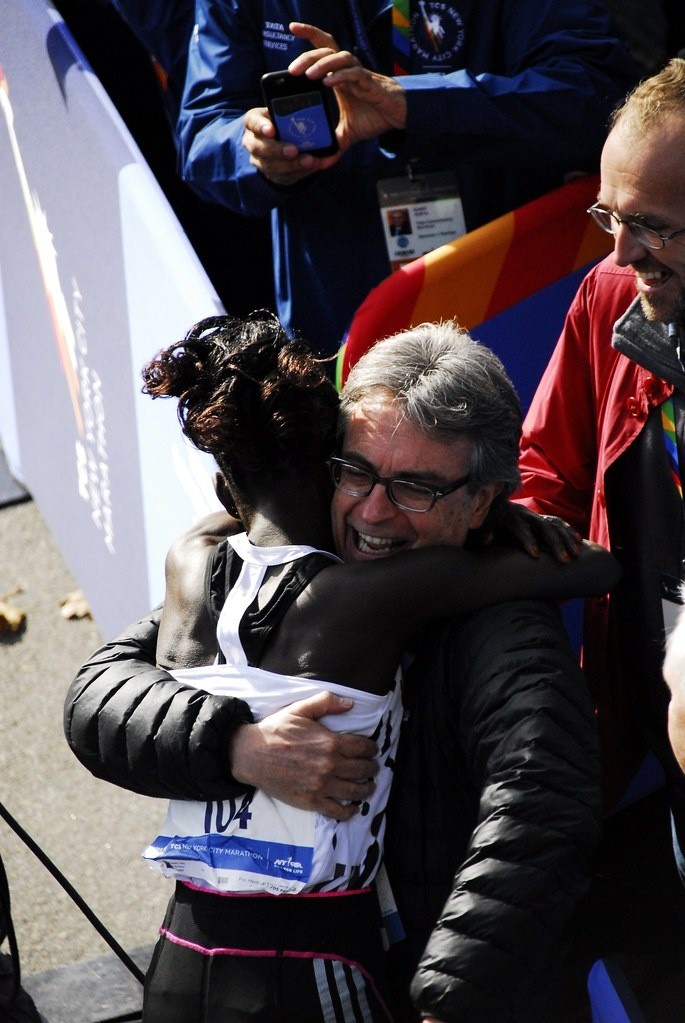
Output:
[389,209,411,235]
[180,0,640,371]
[508,57,685,641]
[661,582,685,774]
[66,308,617,1023]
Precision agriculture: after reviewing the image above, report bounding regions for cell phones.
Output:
[261,70,340,160]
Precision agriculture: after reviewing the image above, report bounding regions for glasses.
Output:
[324,456,475,513]
[586,200,685,249]
